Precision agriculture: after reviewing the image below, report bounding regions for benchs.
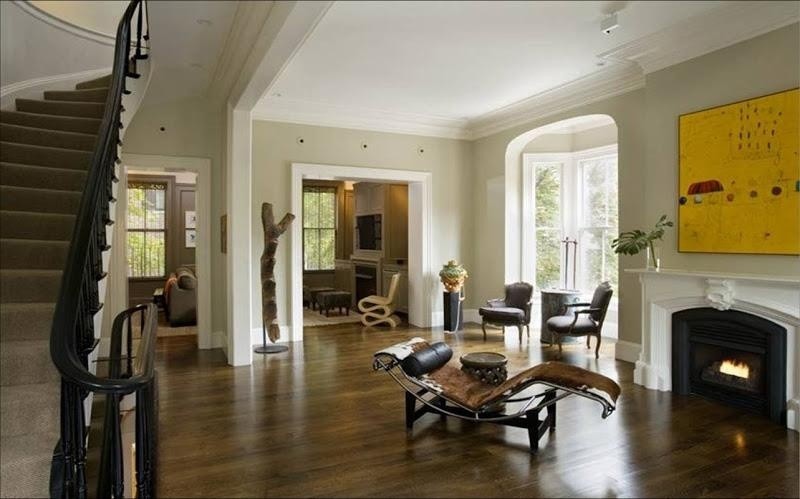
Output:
[153,285,164,308]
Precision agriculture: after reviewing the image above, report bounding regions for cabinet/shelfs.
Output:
[334,259,354,312]
[351,183,408,216]
[382,263,409,315]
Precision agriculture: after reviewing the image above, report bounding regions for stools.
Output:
[459,350,508,385]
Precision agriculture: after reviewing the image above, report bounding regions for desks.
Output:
[540,289,582,344]
[316,290,352,316]
[304,285,334,311]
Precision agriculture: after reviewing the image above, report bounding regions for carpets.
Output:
[132,302,198,338]
[304,304,363,328]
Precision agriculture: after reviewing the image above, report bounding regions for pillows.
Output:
[401,340,453,375]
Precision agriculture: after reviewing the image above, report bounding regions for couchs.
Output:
[161,261,197,325]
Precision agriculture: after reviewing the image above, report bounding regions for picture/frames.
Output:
[677,87,800,256]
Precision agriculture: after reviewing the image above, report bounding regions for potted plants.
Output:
[610,213,675,271]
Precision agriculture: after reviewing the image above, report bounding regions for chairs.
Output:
[478,282,536,340]
[549,280,613,355]
[371,334,623,458]
[358,272,405,330]
